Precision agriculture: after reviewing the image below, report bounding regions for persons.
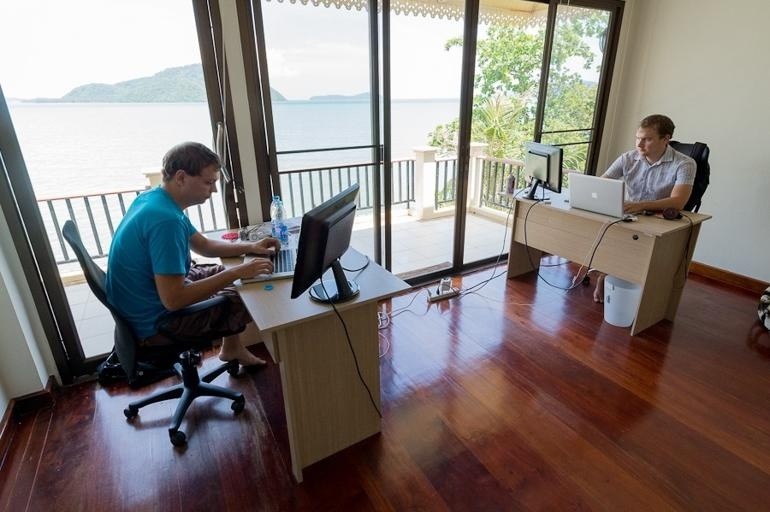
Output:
[105,141,280,364]
[593,115,697,303]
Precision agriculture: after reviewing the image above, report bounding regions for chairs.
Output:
[61,218,246,452]
[578,139,710,288]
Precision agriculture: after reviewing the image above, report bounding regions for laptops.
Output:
[568,172,630,219]
[240,245,298,284]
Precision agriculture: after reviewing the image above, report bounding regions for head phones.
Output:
[663,206,683,220]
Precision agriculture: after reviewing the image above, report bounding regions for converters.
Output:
[440,277,452,291]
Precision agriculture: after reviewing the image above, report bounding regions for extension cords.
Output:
[427,287,460,301]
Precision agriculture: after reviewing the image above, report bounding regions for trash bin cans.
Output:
[604,275,642,327]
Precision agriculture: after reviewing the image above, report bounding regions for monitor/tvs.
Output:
[291,183,360,302]
[518,141,563,200]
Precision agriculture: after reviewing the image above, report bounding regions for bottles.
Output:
[269,195,284,239]
[272,202,289,247]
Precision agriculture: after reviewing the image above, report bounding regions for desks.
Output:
[504,186,712,338]
[214,216,415,482]
